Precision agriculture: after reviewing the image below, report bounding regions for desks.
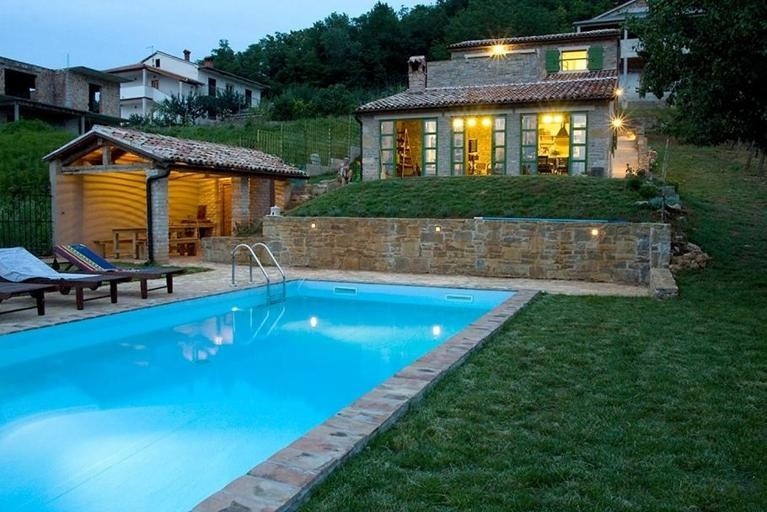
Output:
[112,223,214,258]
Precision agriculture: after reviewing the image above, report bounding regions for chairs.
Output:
[54,243,185,300]
[554,159,566,175]
[0,284,54,316]
[486,163,491,175]
[538,155,552,175]
[0,247,129,310]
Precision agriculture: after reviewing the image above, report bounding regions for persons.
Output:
[335,157,353,185]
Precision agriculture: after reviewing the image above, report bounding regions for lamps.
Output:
[555,122,569,138]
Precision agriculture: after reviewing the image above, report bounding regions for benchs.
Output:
[94,237,145,257]
[137,238,199,260]
